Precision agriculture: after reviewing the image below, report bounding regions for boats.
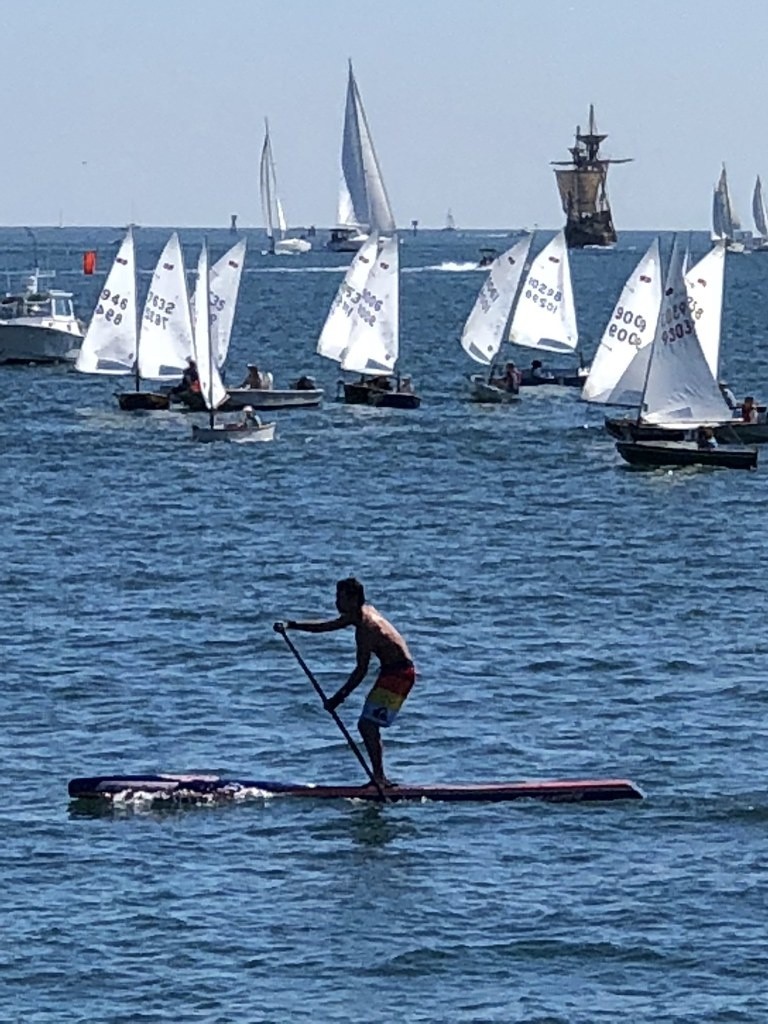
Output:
[0,243,87,366]
[549,101,635,248]
[476,247,497,267]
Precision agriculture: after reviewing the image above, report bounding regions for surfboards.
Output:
[65,770,648,808]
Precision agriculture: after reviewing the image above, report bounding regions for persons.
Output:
[241,406,264,429]
[399,373,415,394]
[497,361,522,395]
[699,430,719,449]
[280,579,417,792]
[742,395,758,423]
[181,359,201,388]
[529,359,543,376]
[242,364,264,389]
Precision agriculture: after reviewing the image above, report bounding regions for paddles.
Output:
[272,620,387,802]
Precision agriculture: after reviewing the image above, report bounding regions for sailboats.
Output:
[329,55,397,252]
[256,116,313,256]
[507,227,588,387]
[459,229,535,405]
[577,161,768,474]
[73,223,423,444]
